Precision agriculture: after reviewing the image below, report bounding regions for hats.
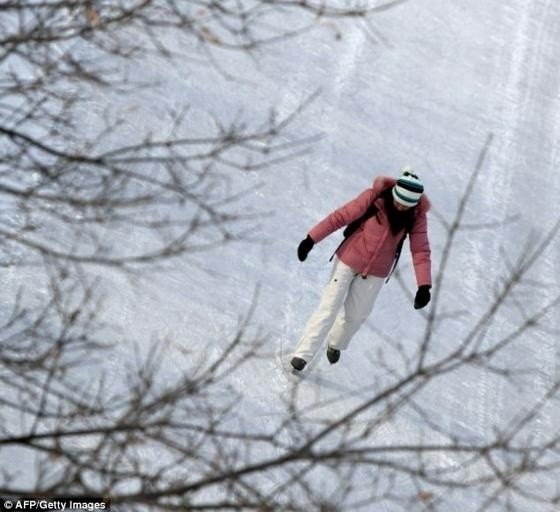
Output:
[392,167,424,207]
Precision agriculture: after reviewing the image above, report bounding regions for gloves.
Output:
[298,234,315,262]
[414,285,432,309]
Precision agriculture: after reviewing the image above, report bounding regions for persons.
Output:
[286,165,433,371]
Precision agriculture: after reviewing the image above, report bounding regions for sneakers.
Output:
[327,344,340,364]
[290,357,307,370]
